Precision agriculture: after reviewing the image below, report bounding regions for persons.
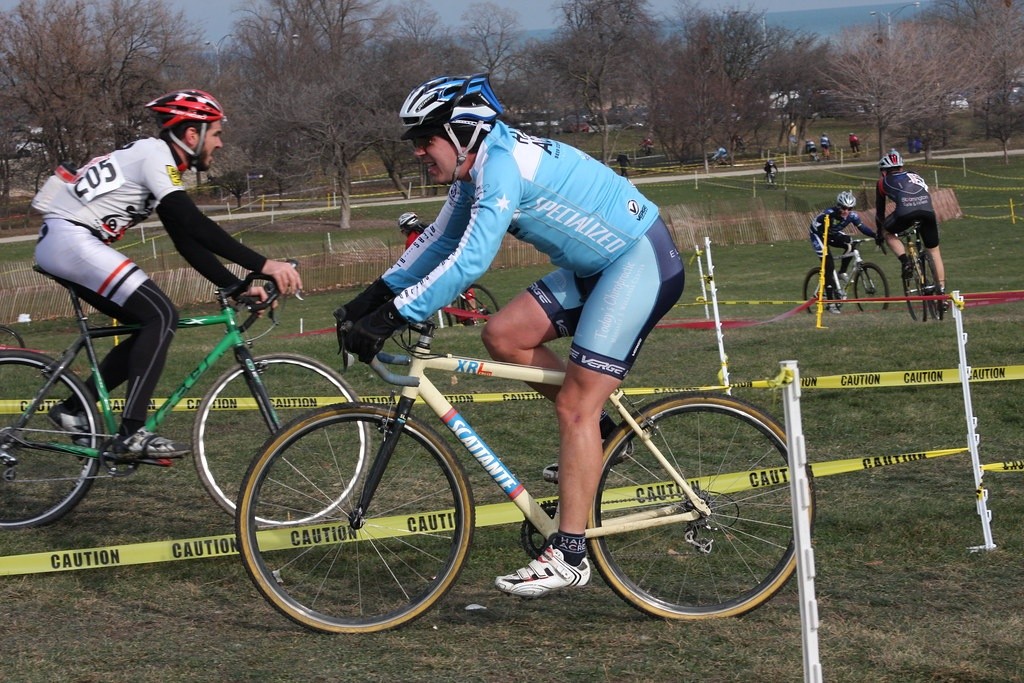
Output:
[639,137,654,154]
[735,135,743,146]
[907,136,922,153]
[820,133,831,157]
[764,160,778,183]
[809,192,875,314]
[805,139,819,159]
[333,72,686,598]
[875,149,950,311]
[849,133,860,153]
[615,152,631,176]
[31,89,302,459]
[398,212,479,320]
[712,145,728,165]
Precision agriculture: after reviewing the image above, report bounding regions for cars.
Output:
[15,127,88,158]
[784,94,874,120]
[561,114,643,134]
[951,94,969,110]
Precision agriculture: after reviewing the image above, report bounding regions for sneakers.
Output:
[825,303,841,315]
[837,271,854,284]
[494,544,592,597]
[543,435,633,482]
[46,399,91,453]
[113,426,191,460]
[901,261,913,280]
[937,301,948,313]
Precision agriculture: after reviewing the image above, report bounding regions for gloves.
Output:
[344,296,407,356]
[874,233,880,245]
[876,230,885,240]
[336,274,394,342]
[840,235,850,244]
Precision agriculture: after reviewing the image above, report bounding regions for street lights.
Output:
[869,1,921,39]
[732,11,770,132]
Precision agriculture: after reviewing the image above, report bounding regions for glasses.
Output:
[401,229,404,233]
[413,130,437,148]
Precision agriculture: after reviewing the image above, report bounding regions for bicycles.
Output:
[764,172,778,190]
[803,237,890,311]
[235,307,816,636]
[878,228,944,322]
[0,256,372,529]
[444,284,499,328]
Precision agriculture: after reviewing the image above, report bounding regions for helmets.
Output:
[398,211,420,228]
[837,190,857,208]
[400,71,505,143]
[878,148,904,168]
[145,88,225,129]
